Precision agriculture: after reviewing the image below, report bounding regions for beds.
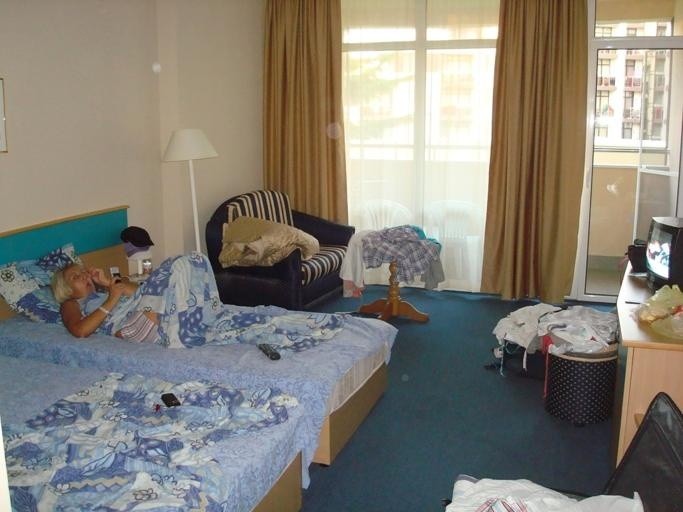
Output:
[0,206,398,467]
[0,353,319,512]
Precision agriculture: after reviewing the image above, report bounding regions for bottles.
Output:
[143,259,151,274]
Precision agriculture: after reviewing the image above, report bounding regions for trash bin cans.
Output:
[545,344,618,427]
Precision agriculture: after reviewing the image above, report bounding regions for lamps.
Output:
[163,129,217,253]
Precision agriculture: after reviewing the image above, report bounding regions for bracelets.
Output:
[98,305,110,315]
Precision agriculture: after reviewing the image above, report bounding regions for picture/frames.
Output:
[0,78,9,153]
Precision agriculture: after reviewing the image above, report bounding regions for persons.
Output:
[54,253,205,345]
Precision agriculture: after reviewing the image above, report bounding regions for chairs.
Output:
[442,391,683,512]
[205,190,355,311]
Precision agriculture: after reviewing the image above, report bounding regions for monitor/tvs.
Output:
[645,216,683,296]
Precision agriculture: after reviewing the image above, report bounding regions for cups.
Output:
[627,239,647,271]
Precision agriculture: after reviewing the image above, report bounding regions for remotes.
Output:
[259,344,281,360]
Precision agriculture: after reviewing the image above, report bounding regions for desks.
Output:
[616,258,683,467]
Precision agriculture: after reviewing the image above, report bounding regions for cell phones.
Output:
[161,393,181,408]
[109,267,121,283]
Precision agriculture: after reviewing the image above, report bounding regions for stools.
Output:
[358,240,441,322]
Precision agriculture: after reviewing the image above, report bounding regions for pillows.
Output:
[0,243,98,326]
